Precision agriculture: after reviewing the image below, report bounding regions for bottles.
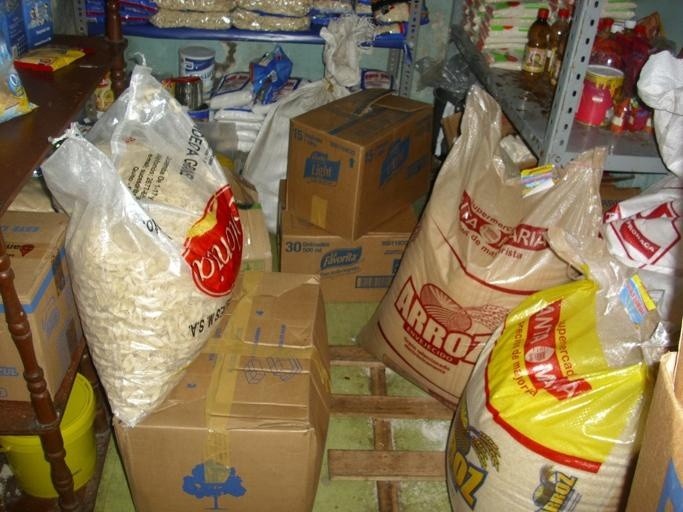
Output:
[521,6,645,91]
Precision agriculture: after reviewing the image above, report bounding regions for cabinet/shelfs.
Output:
[430,0,683,201]
[0,2,124,512]
[72,0,424,162]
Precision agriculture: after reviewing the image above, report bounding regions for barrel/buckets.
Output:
[1,370,98,502]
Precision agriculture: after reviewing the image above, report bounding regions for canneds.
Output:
[574,64,625,130]
